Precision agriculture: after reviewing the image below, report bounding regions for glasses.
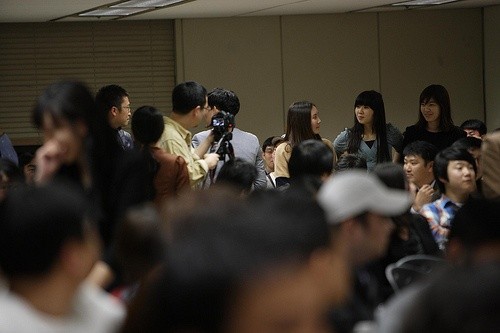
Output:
[121,105,133,112]
[204,106,211,111]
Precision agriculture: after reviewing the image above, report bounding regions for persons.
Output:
[273,100,335,187]
[156,80,220,190]
[125,105,189,194]
[0,120,500,333]
[395,84,468,167]
[334,90,404,175]
[33,78,135,235]
[191,87,269,193]
[96,83,133,164]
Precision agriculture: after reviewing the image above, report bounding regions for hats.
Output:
[271,134,287,145]
[316,168,412,225]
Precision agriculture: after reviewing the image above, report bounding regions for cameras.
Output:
[211,110,235,143]
